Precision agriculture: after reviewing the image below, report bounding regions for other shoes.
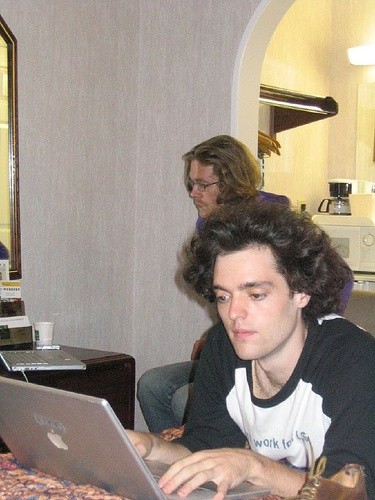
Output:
[295,460,370,500]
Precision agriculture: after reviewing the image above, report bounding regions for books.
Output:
[0,259,32,346]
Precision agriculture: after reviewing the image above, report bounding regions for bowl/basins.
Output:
[349,194,375,216]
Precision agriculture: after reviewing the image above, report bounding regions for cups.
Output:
[34,322,55,347]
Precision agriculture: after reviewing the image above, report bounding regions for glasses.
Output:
[187,176,222,192]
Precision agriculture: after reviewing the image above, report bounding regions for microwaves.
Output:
[312,214,375,273]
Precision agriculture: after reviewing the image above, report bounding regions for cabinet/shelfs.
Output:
[0,345,135,454]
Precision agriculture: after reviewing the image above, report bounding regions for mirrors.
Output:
[1,16,23,282]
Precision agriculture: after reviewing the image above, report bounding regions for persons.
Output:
[136,134,355,433]
[125,198,375,500]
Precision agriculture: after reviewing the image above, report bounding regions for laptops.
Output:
[0,376,271,500]
[0,349,86,371]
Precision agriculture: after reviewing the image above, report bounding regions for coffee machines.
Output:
[318,179,374,215]
